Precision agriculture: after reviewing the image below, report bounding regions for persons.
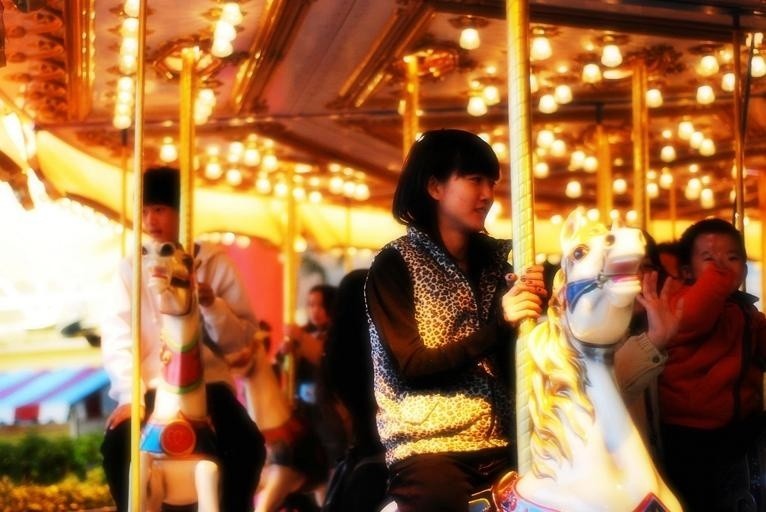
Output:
[95,163,263,511]
[613,217,766,512]
[361,127,550,512]
[250,269,367,511]
